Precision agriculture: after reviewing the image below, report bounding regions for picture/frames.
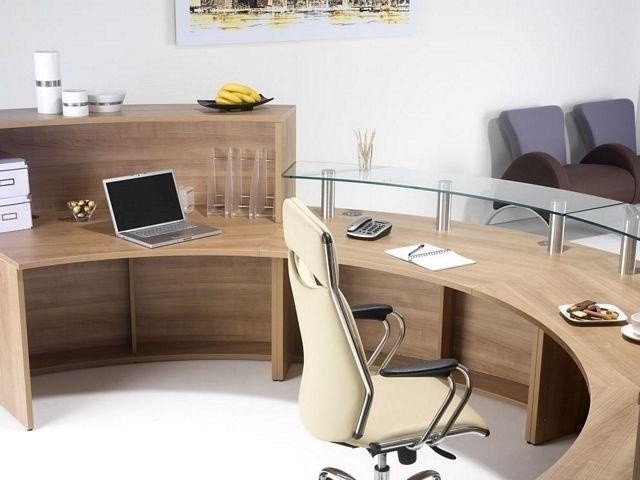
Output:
[173,1,417,43]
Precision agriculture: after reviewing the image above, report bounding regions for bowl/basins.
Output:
[88,88,126,113]
[66,200,97,222]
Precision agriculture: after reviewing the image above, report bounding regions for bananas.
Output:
[214,82,261,104]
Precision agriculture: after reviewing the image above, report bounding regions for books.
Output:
[384,242,477,272]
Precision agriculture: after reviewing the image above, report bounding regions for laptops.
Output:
[102,168,222,249]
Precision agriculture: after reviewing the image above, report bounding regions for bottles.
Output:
[33,52,62,114]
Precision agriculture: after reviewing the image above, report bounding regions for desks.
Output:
[1,206,638,479]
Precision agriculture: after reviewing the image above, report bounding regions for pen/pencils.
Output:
[409,245,424,255]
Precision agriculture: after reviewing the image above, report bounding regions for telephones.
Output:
[346,216,392,239]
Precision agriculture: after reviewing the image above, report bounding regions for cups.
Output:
[630,313,640,335]
[62,90,89,118]
[358,143,373,173]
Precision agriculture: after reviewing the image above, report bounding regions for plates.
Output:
[621,325,640,342]
[197,94,273,111]
[558,304,628,324]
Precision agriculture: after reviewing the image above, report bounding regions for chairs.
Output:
[283,198,489,479]
[493,100,638,226]
